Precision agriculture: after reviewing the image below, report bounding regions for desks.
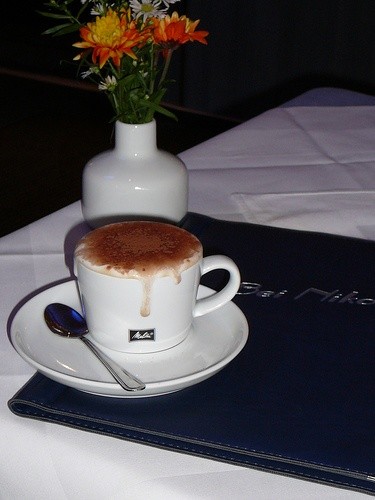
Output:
[0,87,375,500]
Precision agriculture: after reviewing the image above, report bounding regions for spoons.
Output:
[45,301,148,394]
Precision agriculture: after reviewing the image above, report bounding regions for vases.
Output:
[82,119,189,229]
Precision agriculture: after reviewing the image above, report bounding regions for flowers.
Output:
[38,0,210,123]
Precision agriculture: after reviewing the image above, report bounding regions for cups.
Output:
[74,222,240,352]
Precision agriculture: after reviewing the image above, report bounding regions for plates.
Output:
[9,278,249,400]
